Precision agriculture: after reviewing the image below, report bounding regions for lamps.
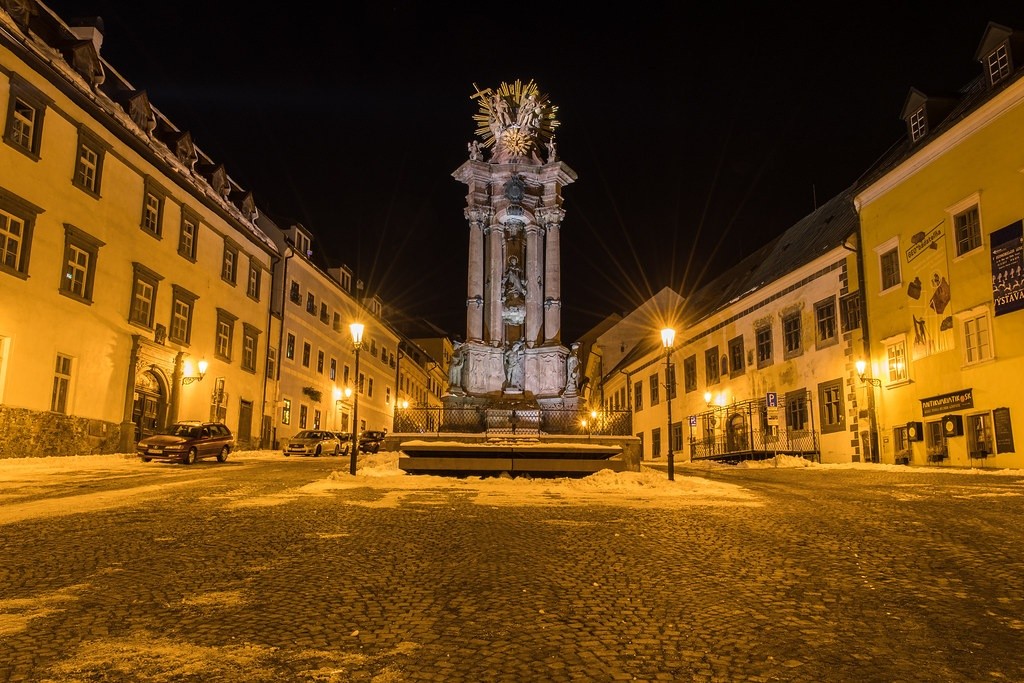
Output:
[704,391,722,412]
[182,355,209,386]
[856,360,882,388]
[336,388,353,406]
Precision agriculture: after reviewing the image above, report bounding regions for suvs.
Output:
[359,430,387,454]
[137,420,234,465]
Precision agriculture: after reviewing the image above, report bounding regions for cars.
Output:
[283,430,340,457]
[332,432,360,456]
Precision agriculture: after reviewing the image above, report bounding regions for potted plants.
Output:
[970,448,987,459]
[927,445,944,462]
[894,450,909,465]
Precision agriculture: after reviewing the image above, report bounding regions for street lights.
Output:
[660,327,676,480]
[349,319,364,476]
